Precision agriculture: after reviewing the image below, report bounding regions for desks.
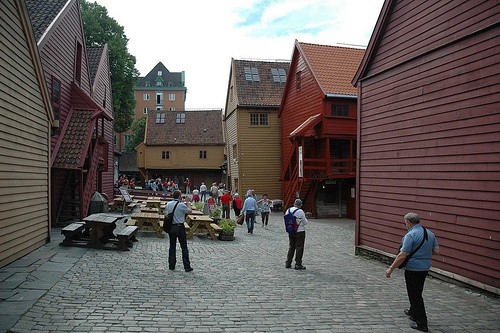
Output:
[187,215,216,240]
[271,199,283,212]
[131,212,163,238]
[141,208,159,213]
[192,210,204,215]
[82,214,117,248]
[147,201,161,207]
[147,197,161,200]
[112,198,128,212]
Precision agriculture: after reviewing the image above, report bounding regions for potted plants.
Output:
[217,218,237,241]
[210,208,223,224]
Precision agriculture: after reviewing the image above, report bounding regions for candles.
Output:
[195,201,203,211]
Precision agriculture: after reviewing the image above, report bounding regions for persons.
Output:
[115,175,273,235]
[165,190,194,271]
[385,213,440,331]
[285,199,307,270]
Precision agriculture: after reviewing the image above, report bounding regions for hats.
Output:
[295,199,302,205]
[225,190,230,192]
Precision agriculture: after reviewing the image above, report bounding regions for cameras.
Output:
[182,196,187,199]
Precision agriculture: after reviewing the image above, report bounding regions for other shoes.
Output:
[404,309,416,321]
[410,323,429,331]
[248,229,250,233]
[185,266,193,272]
[265,226,268,230]
[295,265,306,269]
[251,232,253,234]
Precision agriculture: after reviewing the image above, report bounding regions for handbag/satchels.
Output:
[163,212,173,233]
[397,246,409,269]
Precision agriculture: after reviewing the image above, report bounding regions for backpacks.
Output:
[284,208,303,234]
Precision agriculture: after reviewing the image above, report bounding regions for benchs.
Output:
[61,223,83,245]
[108,200,166,251]
[184,221,221,235]
